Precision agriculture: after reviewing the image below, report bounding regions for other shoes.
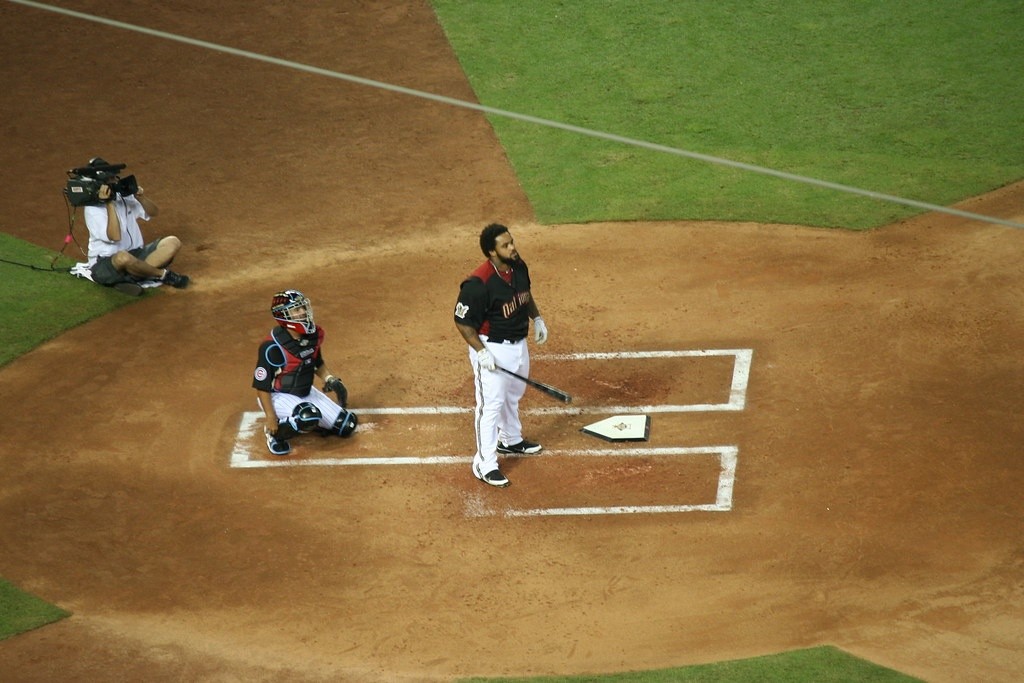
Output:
[115,278,143,297]
[159,271,190,288]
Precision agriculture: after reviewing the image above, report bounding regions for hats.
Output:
[86,157,118,180]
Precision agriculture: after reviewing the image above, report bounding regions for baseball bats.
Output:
[494,363,572,405]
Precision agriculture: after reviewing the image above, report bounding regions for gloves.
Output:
[479,347,497,371]
[534,317,548,345]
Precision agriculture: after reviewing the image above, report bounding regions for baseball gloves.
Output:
[320,376,348,409]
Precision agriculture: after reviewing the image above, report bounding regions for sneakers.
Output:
[498,437,543,455]
[478,468,510,487]
[265,423,290,455]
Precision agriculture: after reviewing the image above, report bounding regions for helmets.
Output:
[269,290,317,334]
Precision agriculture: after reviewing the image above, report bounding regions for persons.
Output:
[84,158,189,297]
[252,288,347,454]
[453,224,548,487]
